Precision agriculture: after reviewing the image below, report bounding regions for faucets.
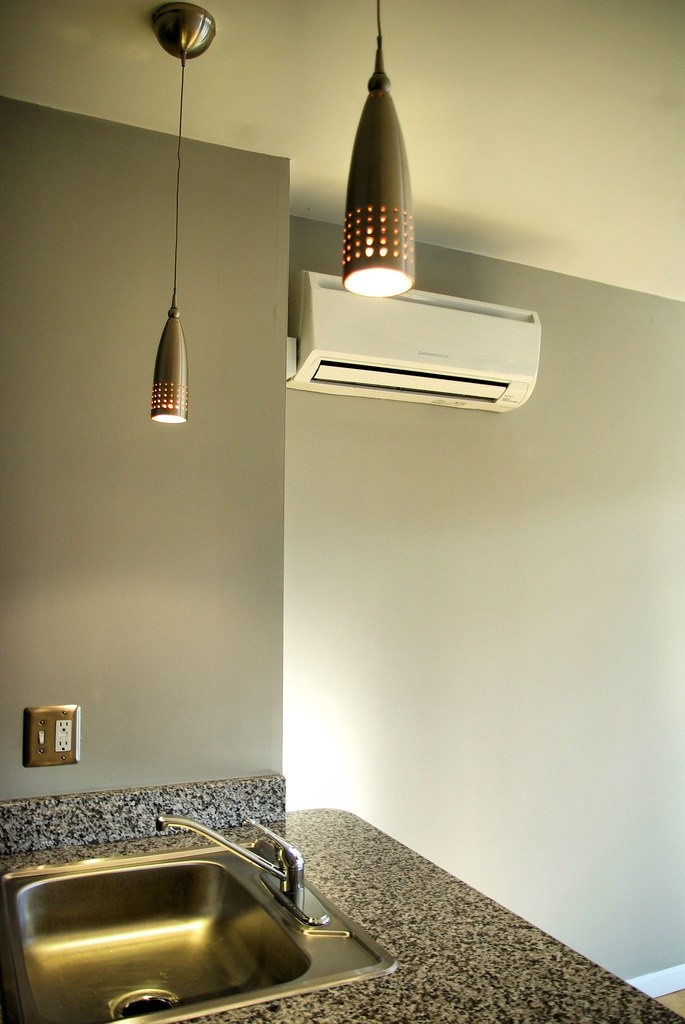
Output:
[155,815,332,929]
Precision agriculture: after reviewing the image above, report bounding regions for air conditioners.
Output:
[287,269,542,414]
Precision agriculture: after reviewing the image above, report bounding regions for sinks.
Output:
[0,835,399,1023]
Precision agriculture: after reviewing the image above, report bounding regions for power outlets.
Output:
[23,705,80,768]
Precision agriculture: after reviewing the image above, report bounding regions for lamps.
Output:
[152,3,214,424]
[343,0,414,298]
